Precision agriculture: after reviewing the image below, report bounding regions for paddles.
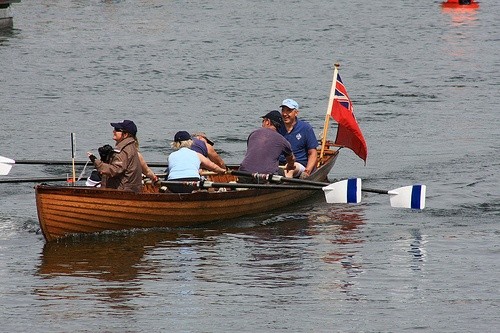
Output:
[0,156,242,175]
[155,178,363,204]
[226,169,427,210]
[0,176,87,183]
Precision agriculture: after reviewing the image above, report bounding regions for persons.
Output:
[87,120,158,189]
[239,110,296,184]
[164,131,226,193]
[280,99,318,178]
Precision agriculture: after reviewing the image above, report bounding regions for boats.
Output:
[442,0,479,8]
[34,132,345,243]
[0,17,13,32]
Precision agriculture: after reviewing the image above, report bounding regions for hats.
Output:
[260,111,284,124]
[191,129,214,147]
[280,99,299,110]
[111,120,137,135]
[175,131,191,142]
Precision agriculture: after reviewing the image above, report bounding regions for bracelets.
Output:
[153,176,157,181]
[304,169,312,174]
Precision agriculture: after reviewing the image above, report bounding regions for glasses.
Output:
[115,128,124,132]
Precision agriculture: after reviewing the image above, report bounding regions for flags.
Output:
[325,65,368,165]
